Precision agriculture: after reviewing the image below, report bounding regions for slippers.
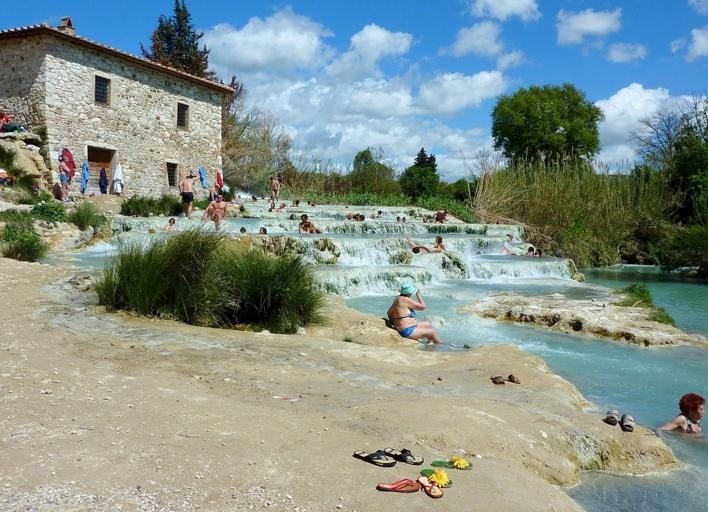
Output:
[622,414,634,432]
[607,410,619,425]
[385,447,424,465]
[417,476,443,498]
[354,450,396,467]
[491,375,506,385]
[377,478,420,492]
[421,468,453,488]
[504,374,520,384]
[432,456,472,469]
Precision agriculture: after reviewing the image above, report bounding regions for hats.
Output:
[398,281,416,295]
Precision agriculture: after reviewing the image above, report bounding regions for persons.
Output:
[166,218,176,231]
[58,155,70,201]
[387,282,442,344]
[0,110,25,132]
[655,394,704,436]
